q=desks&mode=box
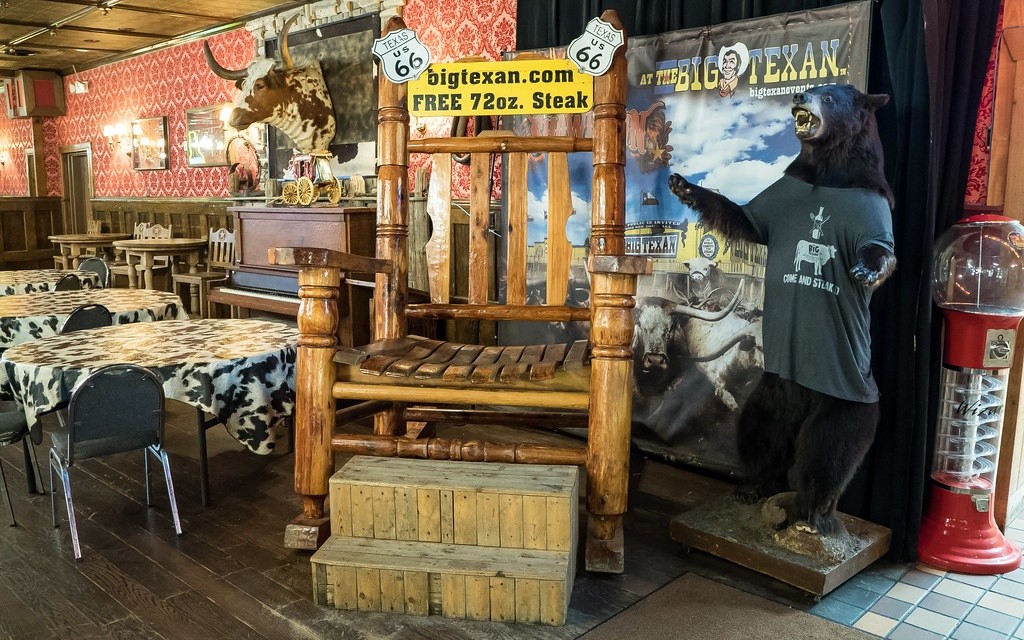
[48,234,132,270]
[112,238,207,317]
[0,288,190,401]
[0,269,104,296]
[2,319,300,507]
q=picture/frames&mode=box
[129,117,170,171]
[185,103,268,167]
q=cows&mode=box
[500,258,765,414]
[203,13,336,151]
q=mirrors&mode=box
[264,11,380,180]
[0,75,38,197]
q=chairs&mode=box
[284,9,637,573]
[171,228,236,318]
[52,219,102,270]
[0,258,182,563]
[109,222,172,291]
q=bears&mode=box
[667,84,896,541]
